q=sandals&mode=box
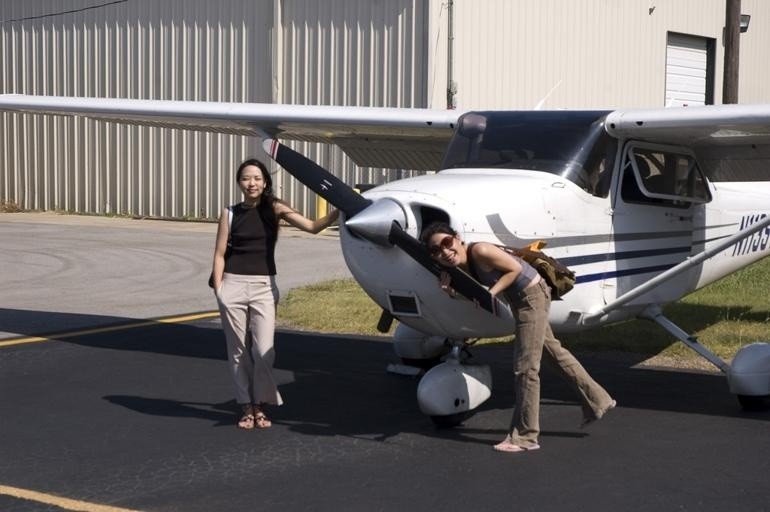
[237,407,273,430]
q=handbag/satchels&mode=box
[207,204,235,288]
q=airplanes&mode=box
[0,97,770,428]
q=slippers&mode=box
[494,437,541,453]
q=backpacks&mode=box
[467,238,577,302]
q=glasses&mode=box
[428,233,456,258]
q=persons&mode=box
[212,158,341,430]
[422,222,617,454]
